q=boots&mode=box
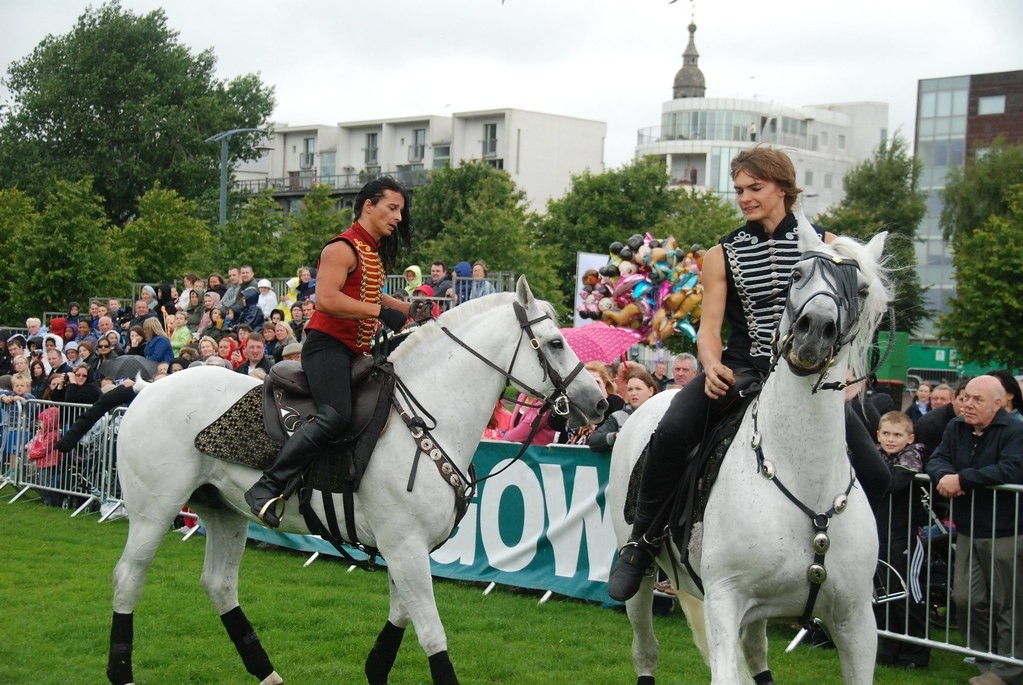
[243,417,328,528]
[608,498,673,602]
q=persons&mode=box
[485,352,1023,685]
[607,147,892,601]
[0,261,495,549]
[750,122,756,142]
[689,166,697,185]
[243,175,429,527]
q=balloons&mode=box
[578,232,707,346]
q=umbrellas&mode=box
[558,321,640,365]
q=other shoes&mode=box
[968,672,1008,685]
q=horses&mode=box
[606,204,909,685]
[106,273,609,685]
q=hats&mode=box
[282,343,303,356]
[65,341,79,352]
[258,279,272,289]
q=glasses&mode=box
[98,345,110,349]
[76,374,88,378]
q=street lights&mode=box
[205,124,276,266]
